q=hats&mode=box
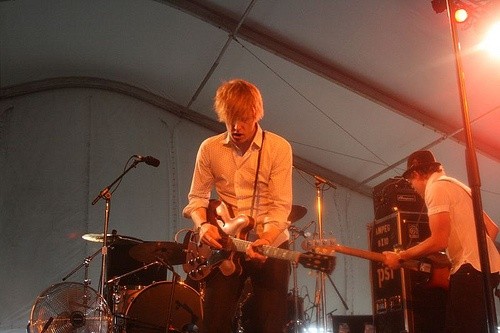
[402,150,442,179]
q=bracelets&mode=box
[396,249,406,263]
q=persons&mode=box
[381,150,500,333]
[182,79,292,333]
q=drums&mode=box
[115,280,204,333]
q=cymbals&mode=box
[287,205,307,223]
[128,240,184,265]
[82,231,144,245]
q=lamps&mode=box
[431,0,447,15]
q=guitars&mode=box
[183,198,338,278]
[299,237,453,288]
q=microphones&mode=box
[315,175,337,189]
[134,155,160,167]
[289,221,313,245]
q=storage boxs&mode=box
[365,208,449,333]
[370,175,435,221]
